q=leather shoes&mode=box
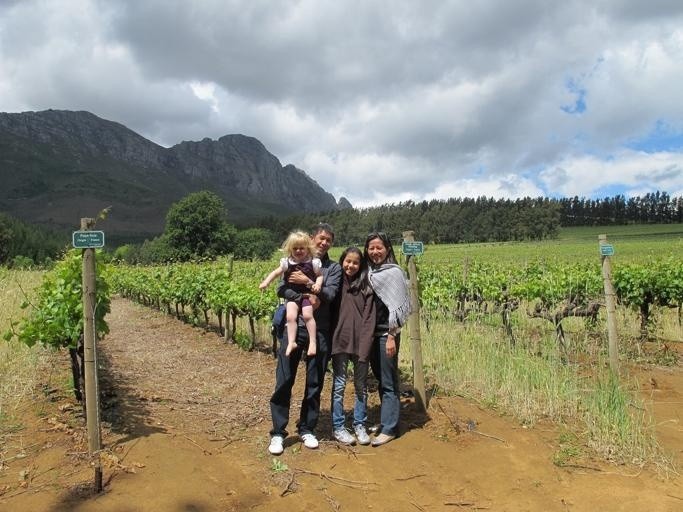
[368,424,397,447]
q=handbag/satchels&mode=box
[272,303,286,339]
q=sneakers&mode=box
[301,433,320,449]
[333,429,356,446]
[268,436,285,455]
[355,425,371,445]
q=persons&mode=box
[330,247,377,445]
[268,222,342,455]
[360,232,412,445]
[259,231,324,357]
